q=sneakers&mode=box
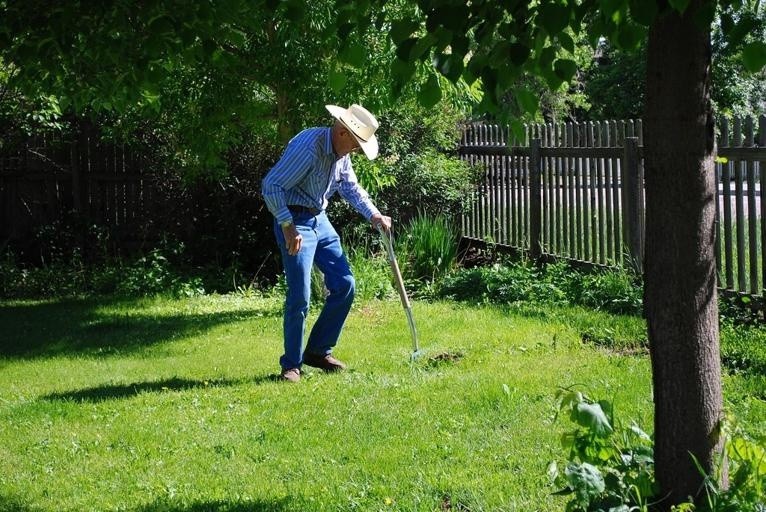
[282,368,301,383]
[302,351,347,371]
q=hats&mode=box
[325,103,380,161]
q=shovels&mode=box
[374,223,420,362]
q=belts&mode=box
[286,204,316,212]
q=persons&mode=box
[261,99,395,382]
